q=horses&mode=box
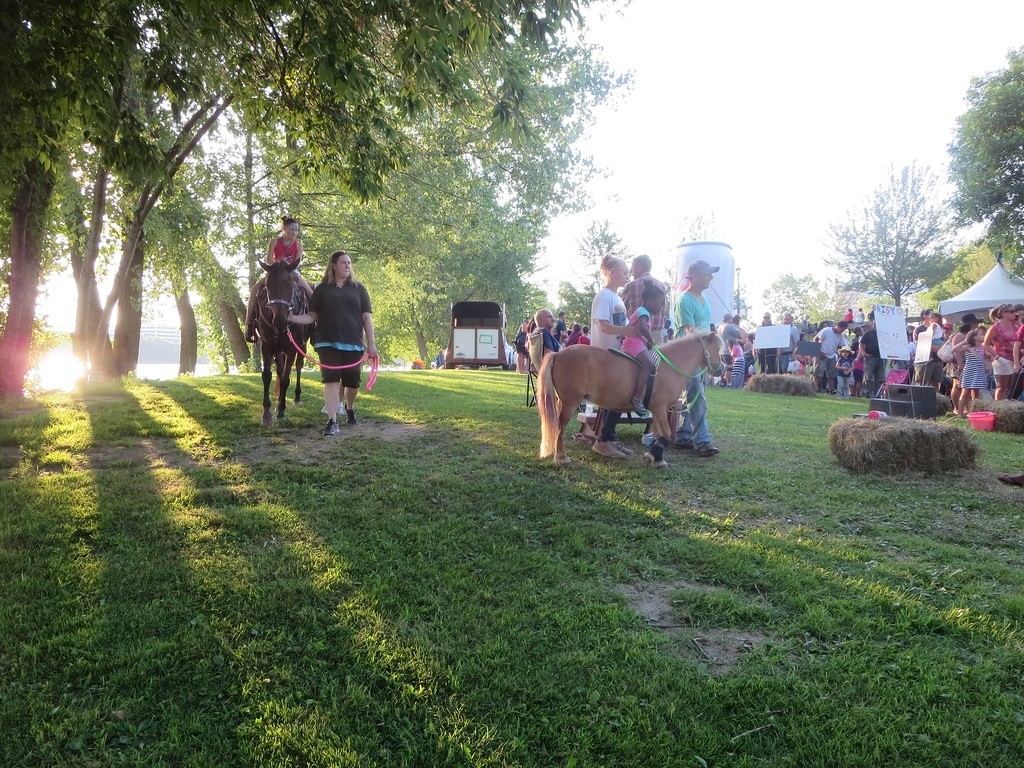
[532,322,725,468]
[248,257,316,426]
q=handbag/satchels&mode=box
[936,338,957,362]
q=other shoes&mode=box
[714,377,751,393]
[814,387,885,398]
[950,408,977,420]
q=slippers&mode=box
[998,475,1023,488]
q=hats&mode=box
[958,313,983,328]
[722,313,733,321]
[1012,303,1024,311]
[685,258,720,278]
[837,345,853,354]
[597,252,623,274]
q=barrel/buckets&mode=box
[967,411,997,432]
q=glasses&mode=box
[1004,310,1015,313]
[923,307,932,314]
[763,316,769,319]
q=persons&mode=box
[949,313,984,416]
[532,310,562,353]
[621,255,666,346]
[984,302,1024,401]
[436,350,444,367]
[513,318,526,372]
[588,254,643,456]
[665,318,716,343]
[245,215,315,326]
[527,317,536,332]
[719,307,952,396]
[622,279,664,417]
[673,261,720,458]
[998,472,1024,487]
[1012,304,1024,322]
[552,311,590,348]
[952,329,989,420]
[1007,323,1024,403]
[287,252,378,436]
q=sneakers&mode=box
[337,401,344,415]
[592,438,631,459]
[320,404,327,415]
[323,419,340,434]
[674,436,694,450]
[696,442,720,456]
[343,405,356,425]
